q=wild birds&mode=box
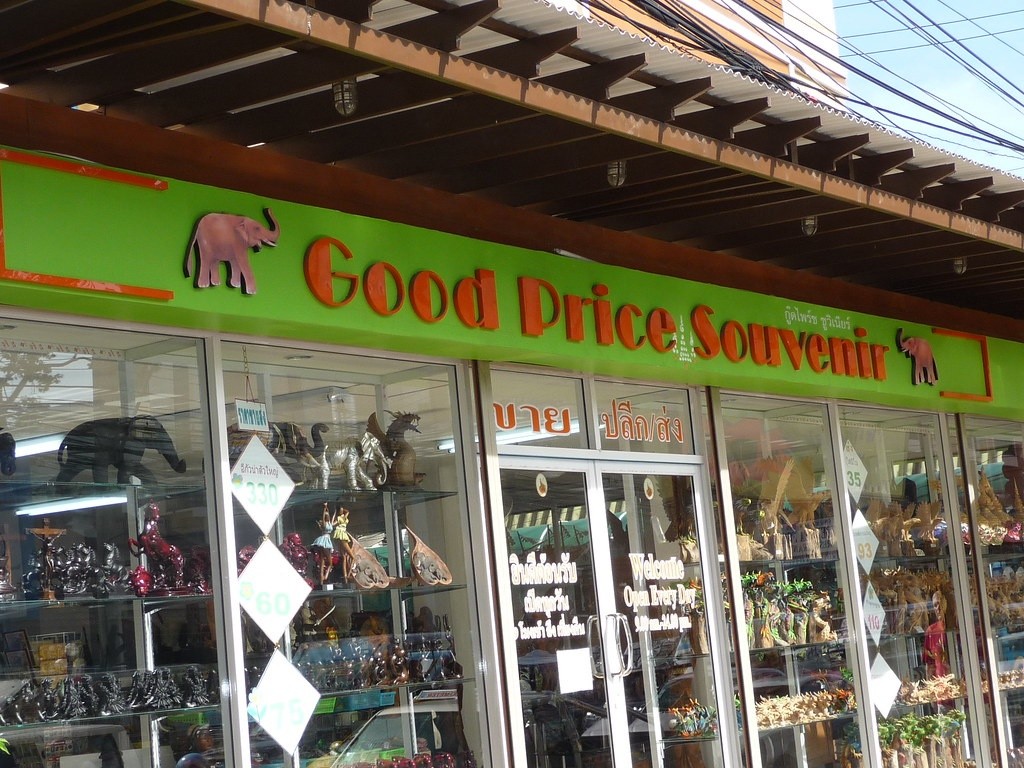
[738,454,945,544]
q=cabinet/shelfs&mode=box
[643,554,1024,768]
[0,483,477,768]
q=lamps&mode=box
[893,450,1003,477]
[14,493,128,516]
[801,216,818,239]
[437,421,606,454]
[154,386,349,421]
[333,77,358,117]
[607,161,626,188]
[954,258,967,274]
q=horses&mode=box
[20,498,213,599]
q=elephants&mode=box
[897,327,939,386]
[184,208,281,295]
[55,414,187,496]
[226,421,391,491]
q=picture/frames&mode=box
[2,629,36,670]
